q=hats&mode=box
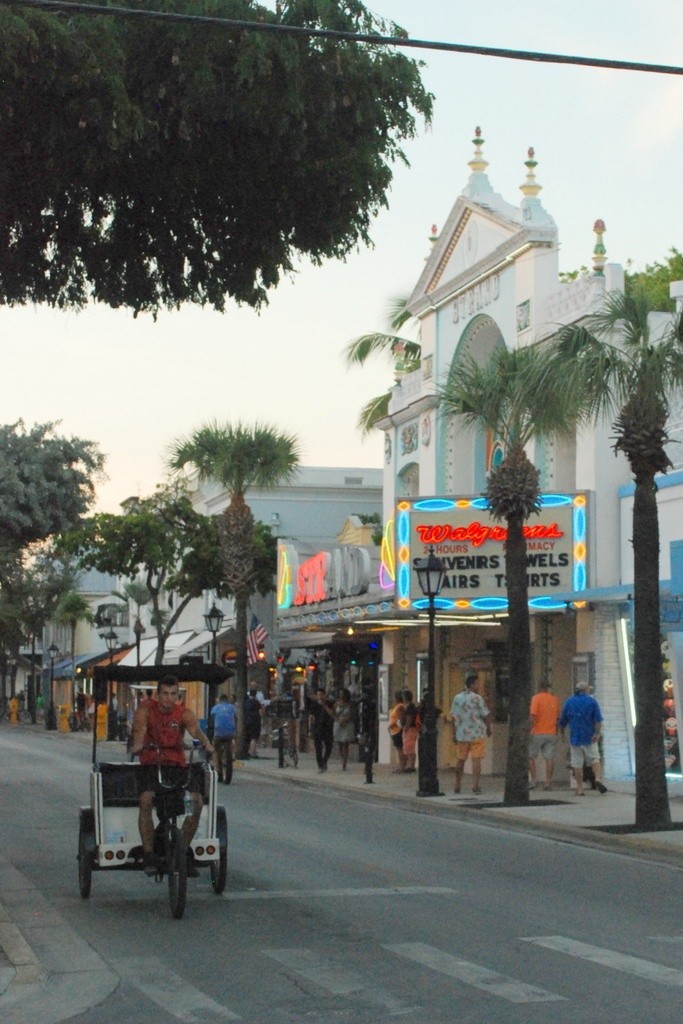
[576,682,593,691]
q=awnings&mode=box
[52,624,233,682]
[279,633,365,648]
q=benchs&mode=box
[99,761,206,807]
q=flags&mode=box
[246,614,268,667]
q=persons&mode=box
[450,675,492,795]
[400,691,416,773]
[528,680,561,790]
[334,688,356,770]
[129,676,215,878]
[387,691,408,774]
[560,681,607,796]
[307,688,336,773]
[14,690,264,759]
[415,687,444,732]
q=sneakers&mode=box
[144,854,156,875]
[217,755,609,796]
[186,857,199,877]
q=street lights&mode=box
[47,643,60,729]
[202,603,227,741]
[411,544,450,797]
[103,624,119,742]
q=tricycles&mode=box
[75,661,236,920]
